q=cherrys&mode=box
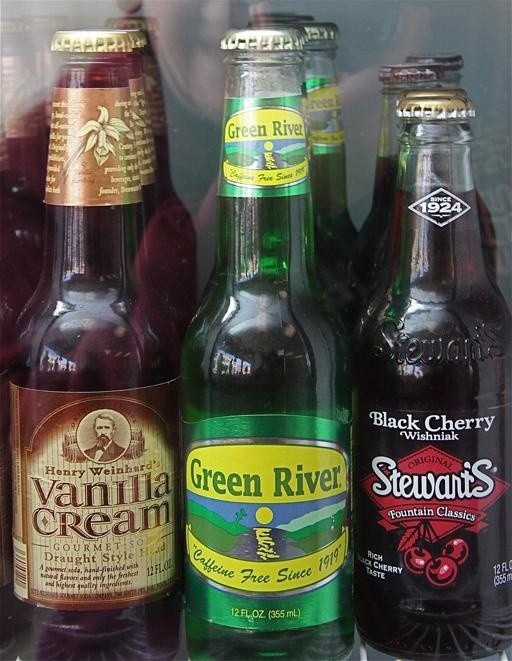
[403,521,469,585]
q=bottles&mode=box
[358,64,441,336]
[1,30,186,661]
[107,16,200,313]
[123,30,185,365]
[358,86,498,351]
[180,25,354,661]
[406,54,497,281]
[253,12,362,319]
[351,94,512,661]
